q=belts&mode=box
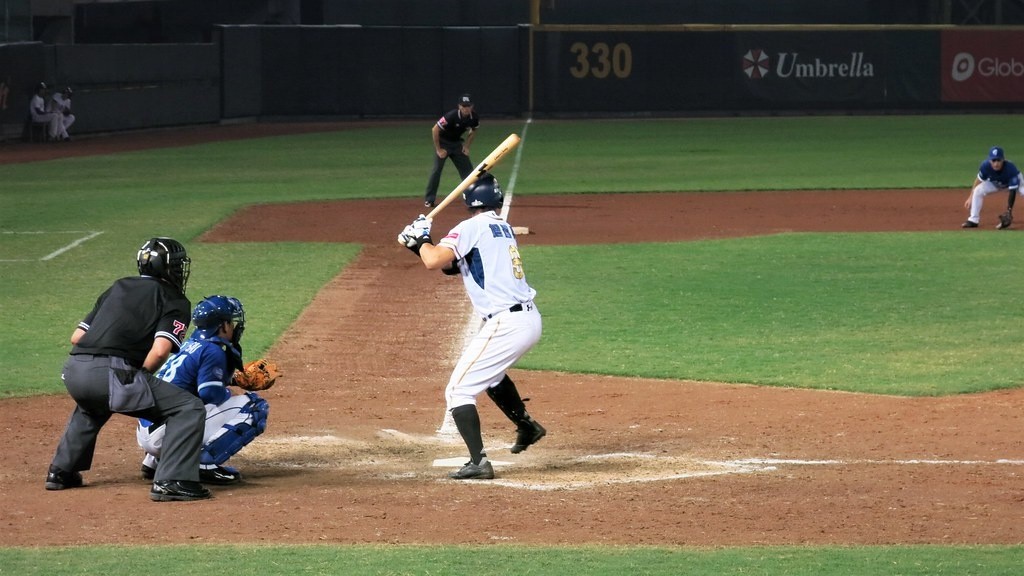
[483,304,529,321]
[137,422,163,434]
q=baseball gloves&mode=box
[235,359,283,391]
[996,210,1012,230]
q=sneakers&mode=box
[46,464,82,490]
[510,420,546,454]
[149,480,210,502]
[199,466,236,485]
[141,464,156,480]
[451,461,494,479]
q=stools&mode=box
[29,121,48,143]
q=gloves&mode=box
[413,213,434,248]
[397,224,420,256]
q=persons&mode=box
[960,146,1024,229]
[29,81,64,144]
[135,295,269,486]
[47,86,76,143]
[45,239,212,502]
[424,95,479,207]
[398,173,547,479]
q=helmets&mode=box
[463,173,503,209]
[137,237,191,296]
[193,296,233,337]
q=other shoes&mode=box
[425,201,434,207]
[961,221,978,228]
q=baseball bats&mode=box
[398,133,521,246]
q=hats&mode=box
[989,147,1004,160]
[458,94,473,107]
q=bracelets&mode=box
[417,234,433,249]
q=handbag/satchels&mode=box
[108,356,156,412]
